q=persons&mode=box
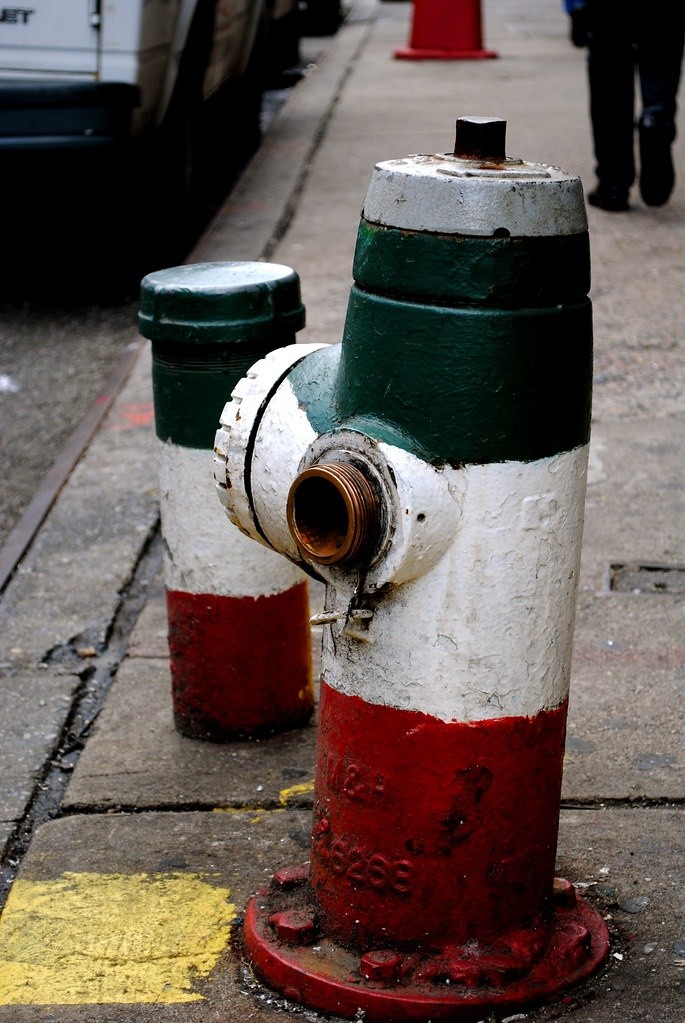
[559,0,685,211]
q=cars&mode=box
[1,1,350,306]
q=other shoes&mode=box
[586,187,631,212]
[640,116,676,208]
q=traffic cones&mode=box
[393,1,502,62]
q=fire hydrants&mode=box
[210,112,610,1023]
